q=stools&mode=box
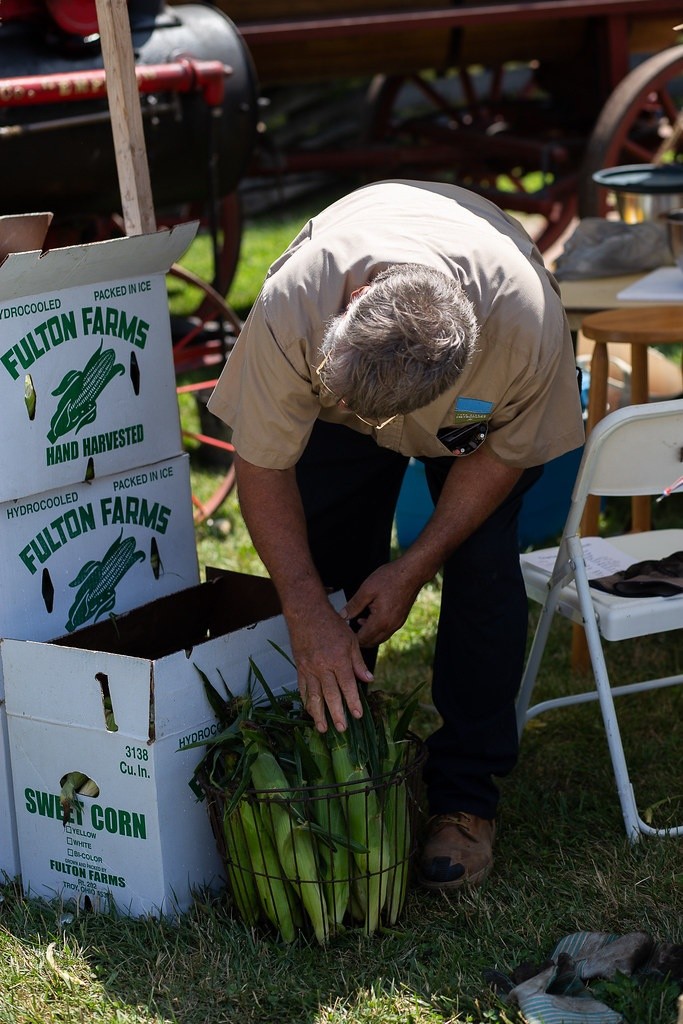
[570,305,683,671]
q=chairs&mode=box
[517,398,683,846]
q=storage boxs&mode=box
[1,209,351,926]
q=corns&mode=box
[60,692,117,796]
[226,703,410,944]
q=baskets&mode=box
[197,729,430,950]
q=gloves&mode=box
[485,930,683,1024]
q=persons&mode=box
[207,178,586,891]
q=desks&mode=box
[554,269,683,359]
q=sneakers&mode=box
[418,810,502,889]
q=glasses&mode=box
[316,346,400,431]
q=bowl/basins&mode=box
[657,208,683,267]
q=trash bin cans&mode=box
[592,163,683,259]
